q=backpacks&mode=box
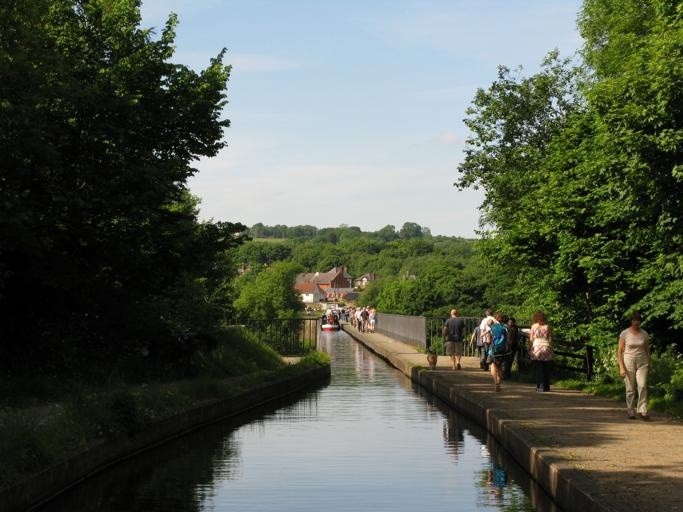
[491,327,507,354]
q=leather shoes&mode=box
[639,414,648,419]
[628,416,634,419]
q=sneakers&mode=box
[456,363,460,370]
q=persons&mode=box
[306,304,377,333]
[470,310,518,392]
[442,309,467,369]
[527,311,554,394]
[618,311,653,421]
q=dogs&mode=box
[427,347,437,371]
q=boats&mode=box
[320,324,338,331]
